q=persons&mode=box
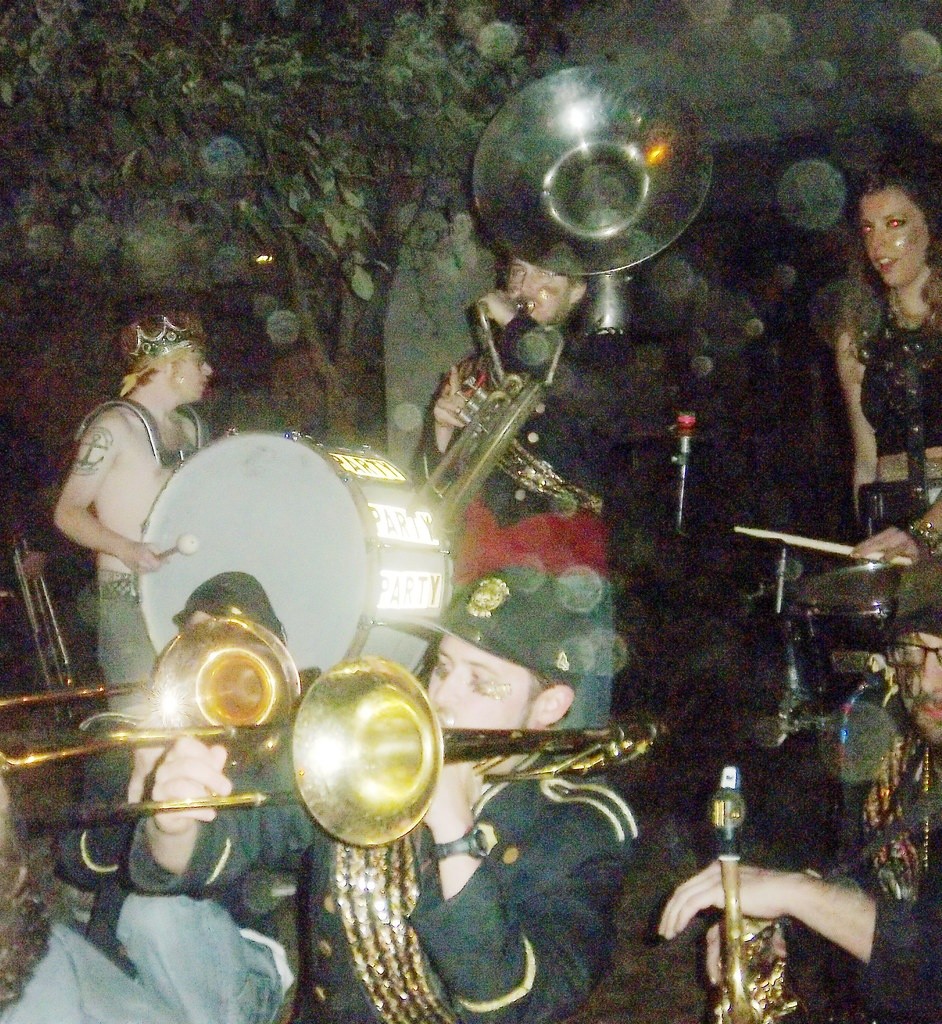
[130,563,639,1024]
[655,607,942,1024]
[52,305,216,724]
[818,164,942,568]
[408,238,629,778]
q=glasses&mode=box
[883,640,940,669]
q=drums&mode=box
[797,557,942,721]
[136,425,452,683]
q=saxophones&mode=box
[710,766,806,1022]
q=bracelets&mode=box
[906,514,942,556]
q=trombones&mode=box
[10,530,82,728]
[0,620,319,776]
[89,657,671,844]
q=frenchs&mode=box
[408,69,720,533]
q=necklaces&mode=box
[888,289,933,327]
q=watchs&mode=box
[428,827,491,861]
[0,570,290,1021]
